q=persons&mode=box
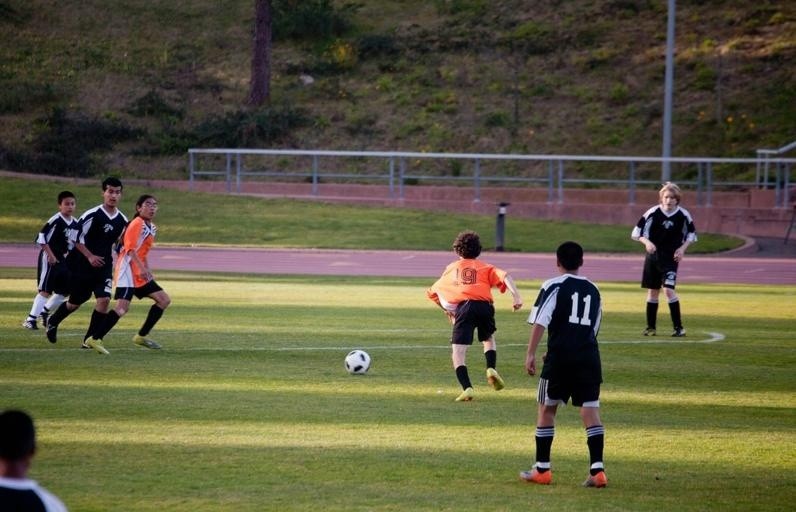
[84,193,170,354]
[44,177,127,347]
[630,181,697,339]
[22,191,77,330]
[0,409,68,512]
[425,229,522,403]
[518,242,609,488]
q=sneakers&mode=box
[671,328,687,337]
[455,387,475,402]
[519,465,553,485]
[82,337,110,356]
[486,368,506,393]
[40,312,48,329]
[22,318,38,331]
[132,333,163,352]
[45,315,58,344]
[642,328,657,337]
[581,470,609,487]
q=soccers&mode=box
[344,350,371,374]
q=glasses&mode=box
[141,204,158,208]
